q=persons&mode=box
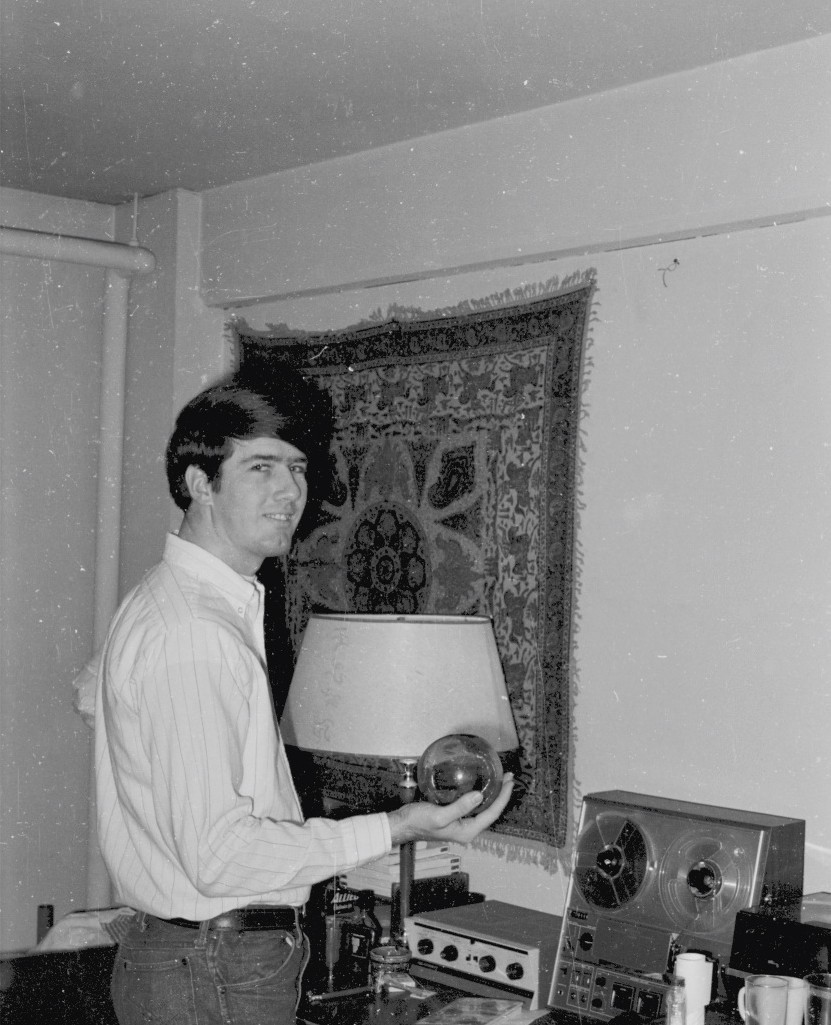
[96,380,513,1025]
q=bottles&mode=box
[664,976,687,1025]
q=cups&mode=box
[738,975,809,1025]
[803,973,831,1025]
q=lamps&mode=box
[278,613,517,945]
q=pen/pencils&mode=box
[312,985,375,1002]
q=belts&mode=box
[157,907,304,931]
